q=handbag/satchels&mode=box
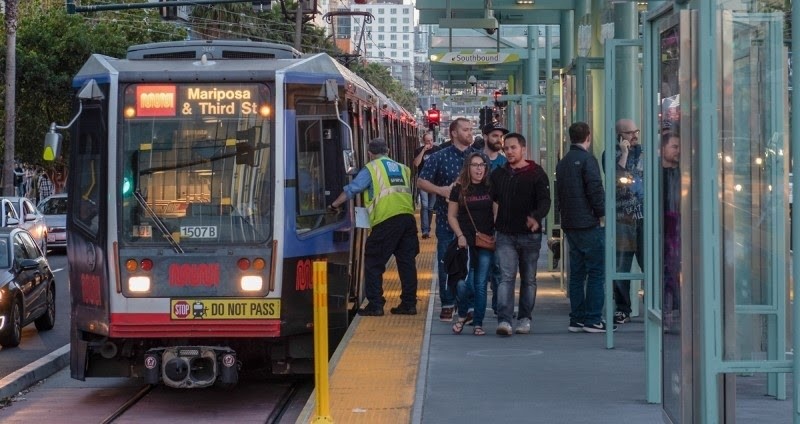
[615,212,637,251]
[476,232,496,251]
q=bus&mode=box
[44,11,420,392]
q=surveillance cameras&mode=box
[483,10,498,35]
[468,75,478,87]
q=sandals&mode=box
[452,319,465,333]
[472,327,486,335]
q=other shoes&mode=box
[583,321,618,332]
[357,303,384,316]
[390,303,417,315]
[614,311,630,324]
[440,307,455,321]
[515,318,531,333]
[496,322,512,335]
[568,321,584,332]
[422,231,429,239]
[465,311,474,325]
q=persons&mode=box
[414,117,553,338]
[601,119,644,325]
[555,121,618,334]
[659,126,680,333]
[327,137,420,317]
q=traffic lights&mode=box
[494,89,507,107]
[428,111,439,123]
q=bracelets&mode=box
[331,204,337,209]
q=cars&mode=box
[0,226,56,348]
[37,193,69,255]
[0,196,48,261]
[716,136,794,253]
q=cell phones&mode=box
[617,134,625,143]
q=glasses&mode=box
[470,162,487,168]
[621,129,640,136]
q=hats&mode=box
[482,122,509,135]
